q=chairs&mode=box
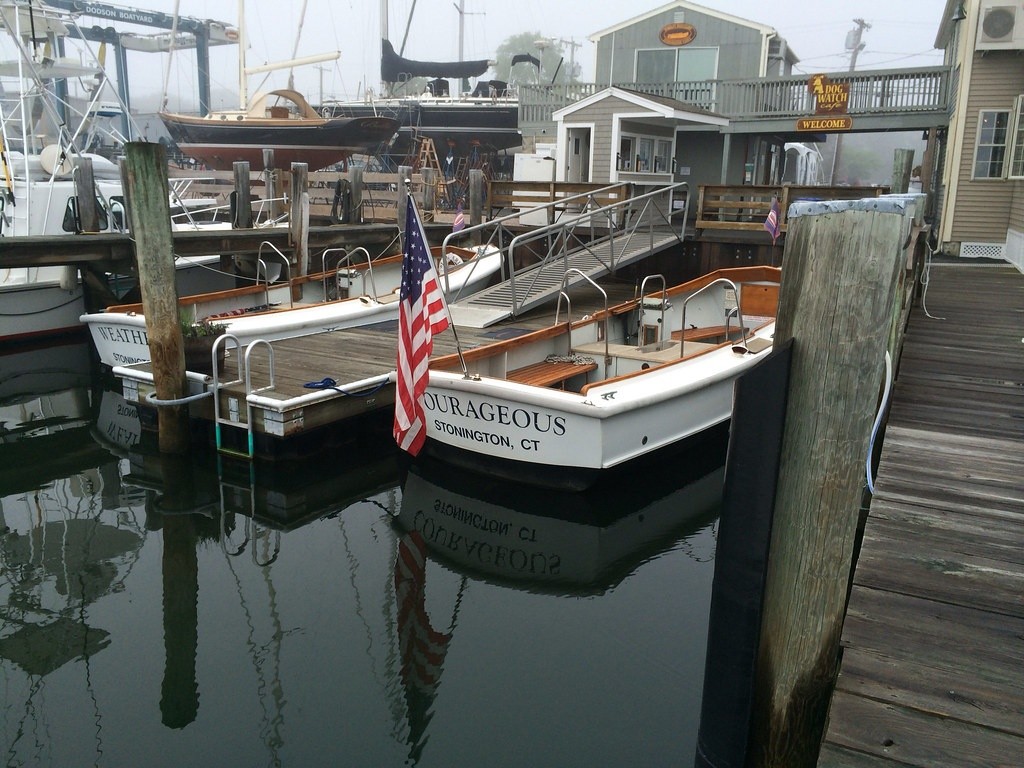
[426,78,449,97]
[471,79,508,98]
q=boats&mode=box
[418,266,783,471]
[0,336,156,679]
[158,0,523,188]
[391,438,727,597]
[82,246,507,402]
[1,0,297,349]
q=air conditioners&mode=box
[974,0,1024,59]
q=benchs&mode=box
[506,360,600,391]
[670,326,750,344]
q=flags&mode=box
[452,204,465,235]
[392,195,451,456]
[764,197,780,244]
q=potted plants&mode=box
[177,305,228,377]
